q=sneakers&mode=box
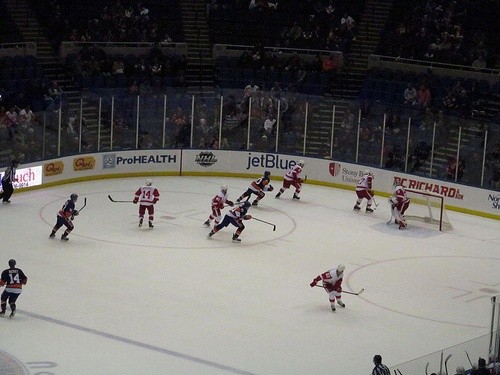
[148,221,154,229]
[137,219,143,227]
[365,207,374,214]
[235,199,240,203]
[0,310,6,318]
[275,192,281,199]
[337,300,345,308]
[206,233,212,240]
[353,204,361,211]
[292,193,300,201]
[8,313,15,320]
[48,233,56,239]
[61,234,69,242]
[252,203,258,207]
[231,233,241,243]
[331,305,336,312]
[204,221,210,227]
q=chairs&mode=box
[0,0,500,184]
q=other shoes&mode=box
[3,200,10,204]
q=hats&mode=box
[244,201,251,207]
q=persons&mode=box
[0,259,27,320]
[397,0,500,72]
[0,82,93,165]
[238,171,274,206]
[276,160,305,200]
[46,0,172,48]
[50,193,79,240]
[207,0,356,51]
[75,47,337,154]
[205,187,234,227]
[394,179,410,229]
[210,202,252,242]
[310,264,345,311]
[470,358,490,375]
[0,159,19,202]
[354,170,374,213]
[372,355,390,375]
[340,68,500,188]
[133,179,160,229]
[456,366,466,375]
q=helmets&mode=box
[8,259,16,267]
[220,185,227,192]
[145,179,153,186]
[298,159,304,165]
[70,194,78,200]
[337,265,345,273]
[264,171,270,177]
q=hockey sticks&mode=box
[424,361,430,375]
[444,353,453,375]
[439,350,444,375]
[251,217,276,232]
[465,349,474,368]
[303,175,308,182]
[223,195,251,207]
[76,197,87,214]
[314,284,365,296]
[4,282,7,285]
[369,188,379,207]
[393,369,397,375]
[396,368,403,375]
[108,195,140,202]
